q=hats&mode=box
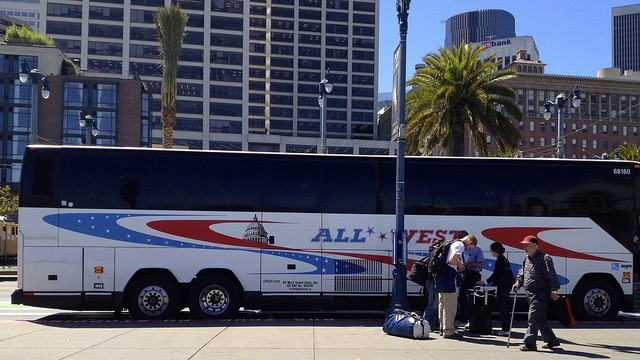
[520,235,538,245]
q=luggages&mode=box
[469,282,492,334]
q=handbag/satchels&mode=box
[406,262,429,286]
[555,295,576,327]
[383,309,430,339]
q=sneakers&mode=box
[542,339,560,348]
[443,332,463,338]
[520,344,537,351]
[497,330,509,336]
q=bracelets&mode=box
[551,289,558,294]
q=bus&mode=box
[12,146,640,321]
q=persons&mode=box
[436,234,479,339]
[457,230,484,327]
[512,236,561,351]
[422,239,443,332]
[476,242,516,336]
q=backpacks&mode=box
[428,239,461,273]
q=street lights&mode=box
[79,111,98,146]
[317,67,334,153]
[18,57,50,145]
[544,85,582,158]
